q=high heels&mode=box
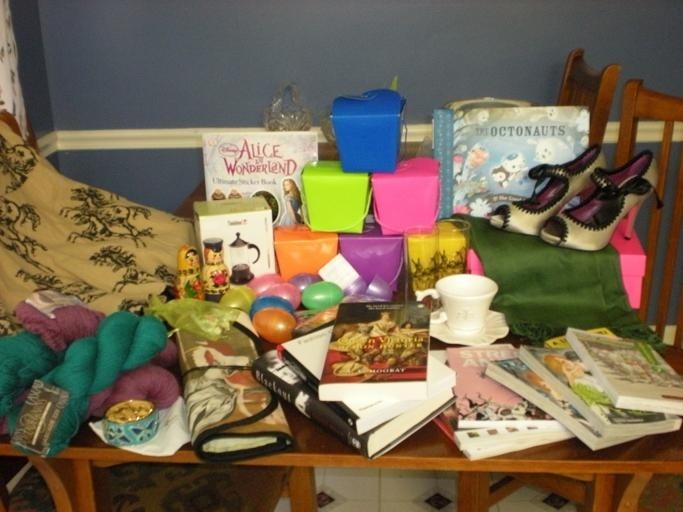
[489,143,606,236]
[539,149,659,252]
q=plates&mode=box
[427,308,509,346]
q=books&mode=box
[202,129,318,227]
[432,105,592,219]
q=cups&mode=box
[437,217,471,277]
[404,226,437,304]
[415,274,498,336]
[227,233,260,286]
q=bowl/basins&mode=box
[101,398,159,444]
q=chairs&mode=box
[380,46,682,373]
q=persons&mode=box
[281,178,305,226]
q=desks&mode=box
[1,401,683,511]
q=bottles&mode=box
[263,79,310,132]
